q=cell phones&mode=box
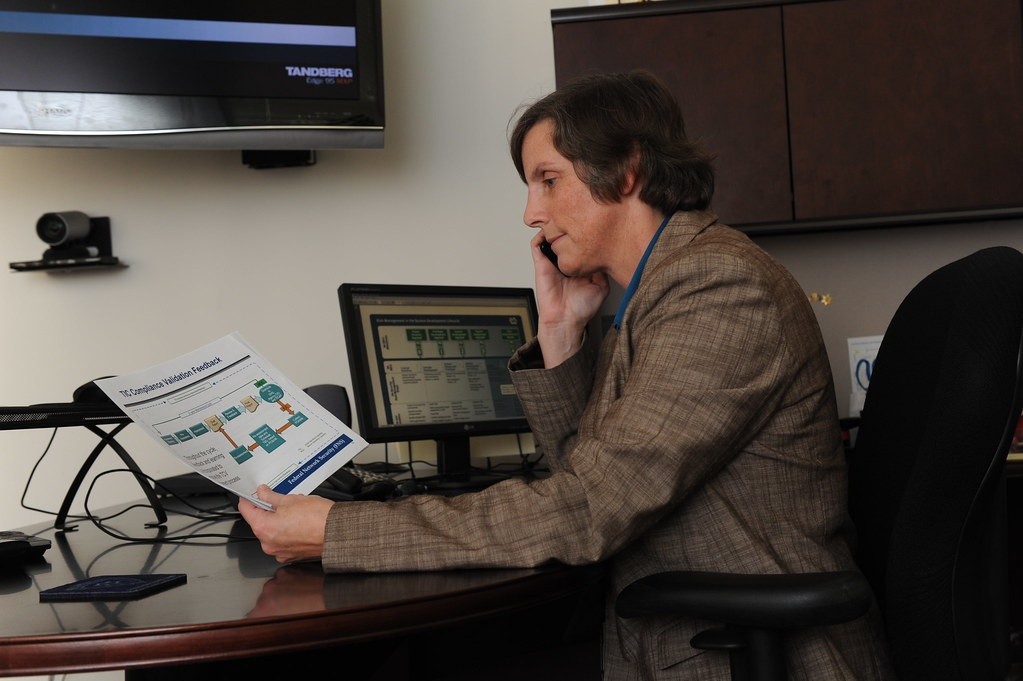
[541,241,569,279]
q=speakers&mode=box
[301,385,352,431]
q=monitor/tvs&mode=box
[340,283,539,497]
[0,0,389,152]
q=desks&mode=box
[0,453,608,681]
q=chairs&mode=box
[615,244,1022,680]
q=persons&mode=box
[238,70,884,680]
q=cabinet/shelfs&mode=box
[551,0,1022,231]
